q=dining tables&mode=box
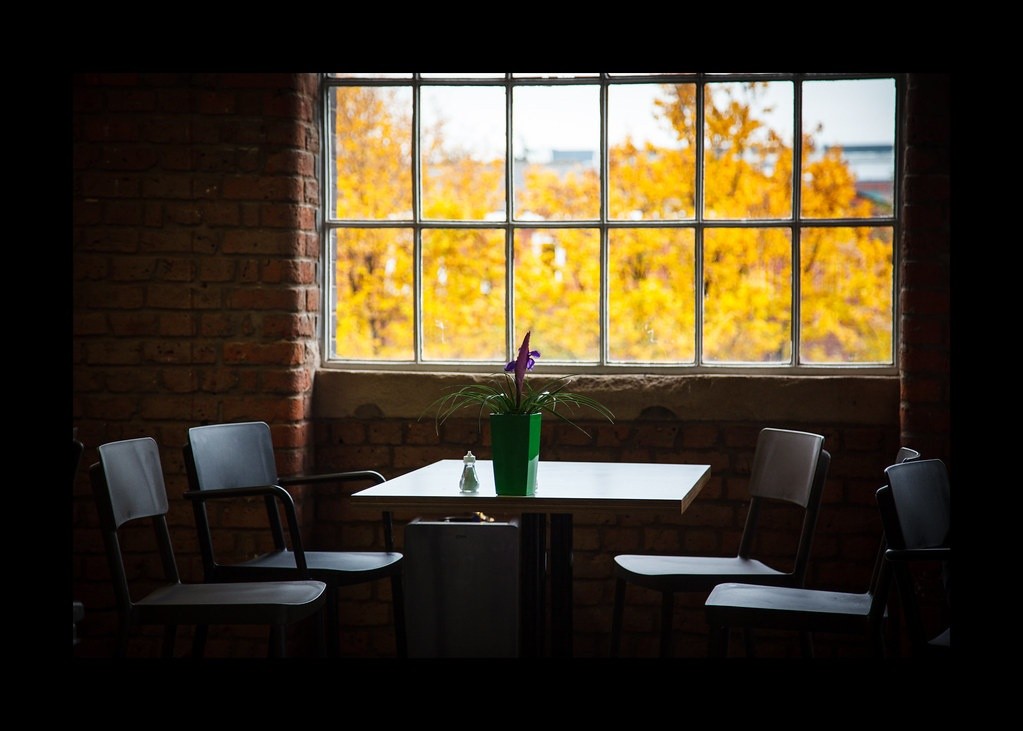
[350,458,712,731]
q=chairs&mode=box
[704,447,921,731]
[875,459,1023,731]
[182,421,410,714]
[608,427,832,717]
[90,438,325,731]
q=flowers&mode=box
[417,331,616,438]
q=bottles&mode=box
[458,450,480,492]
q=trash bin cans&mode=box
[403,514,522,659]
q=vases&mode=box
[490,412,541,496]
